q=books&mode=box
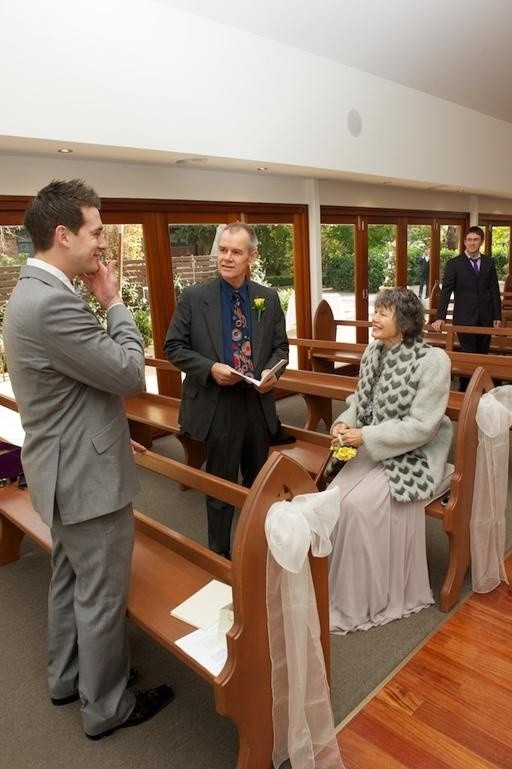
[229,358,287,389]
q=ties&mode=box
[231,289,255,379]
[470,257,480,278]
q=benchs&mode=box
[124,356,495,615]
[275,285,511,428]
[1,392,332,768]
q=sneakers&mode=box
[217,550,231,561]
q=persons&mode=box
[0,177,177,741]
[417,247,431,299]
[430,226,502,394]
[323,285,455,636]
[157,219,291,554]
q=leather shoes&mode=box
[85,683,176,741]
[51,667,140,707]
